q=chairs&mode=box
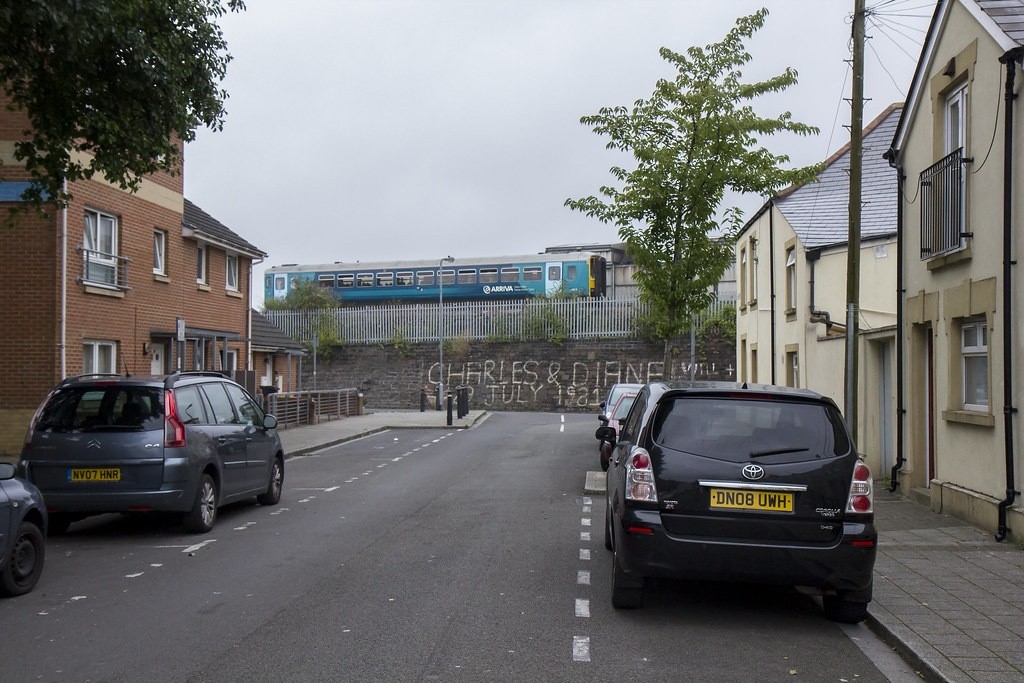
[116,402,144,426]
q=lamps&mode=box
[143,342,150,355]
[264,352,270,366]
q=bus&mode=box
[262,251,608,310]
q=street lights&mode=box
[439,256,456,407]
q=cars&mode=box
[600,384,649,428]
[598,392,639,454]
[0,462,48,594]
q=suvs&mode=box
[595,380,879,626]
[20,373,285,537]
